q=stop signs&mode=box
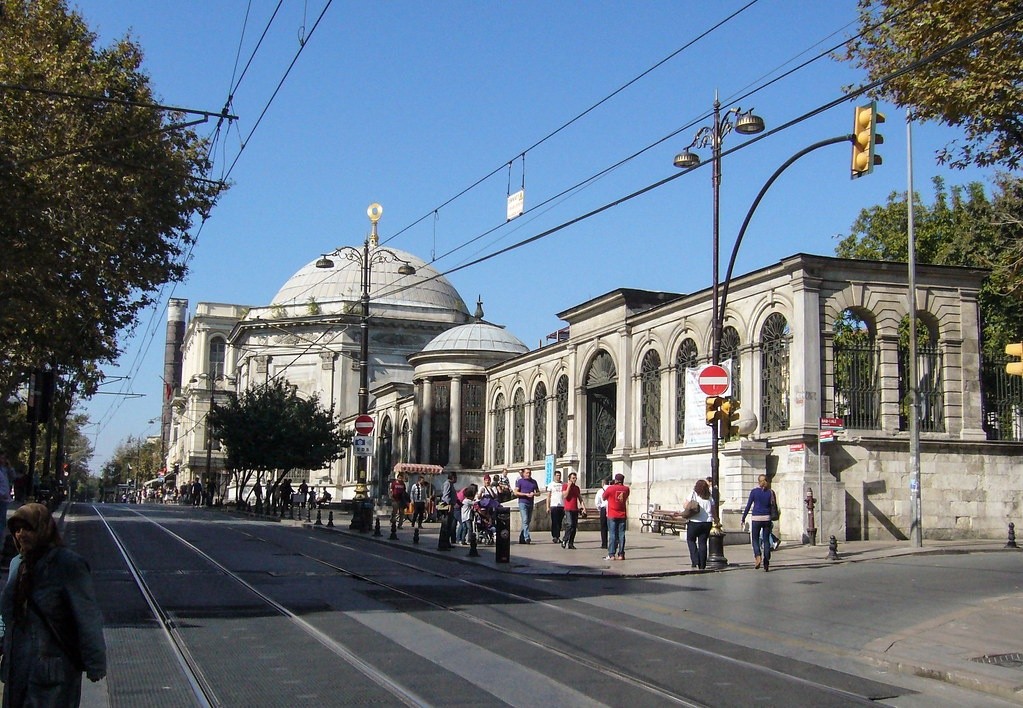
[699,365,730,396]
[354,415,374,436]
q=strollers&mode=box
[473,506,496,545]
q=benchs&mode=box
[576,507,601,530]
[316,497,332,509]
[639,510,688,536]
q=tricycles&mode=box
[394,463,447,523]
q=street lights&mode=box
[316,233,417,532]
[673,89,764,569]
[147,413,179,504]
[189,367,237,511]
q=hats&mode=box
[484,474,491,482]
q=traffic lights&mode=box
[1006,341,1023,378]
[850,101,885,180]
[705,396,722,424]
[719,396,741,442]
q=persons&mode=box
[120,478,217,510]
[410,477,436,529]
[682,481,717,569]
[266,479,296,510]
[298,479,309,509]
[254,480,263,504]
[546,471,565,543]
[595,479,609,548]
[560,472,586,549]
[482,465,483,469]
[452,466,512,546]
[0,503,107,708]
[390,475,406,531]
[705,477,712,494]
[601,473,630,560]
[0,451,12,580]
[514,468,541,544]
[307,485,316,509]
[438,472,464,550]
[740,474,779,573]
[319,486,328,506]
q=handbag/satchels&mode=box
[435,501,451,522]
[770,490,779,521]
[682,492,700,518]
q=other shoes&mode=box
[552,537,562,544]
[519,539,531,544]
[561,540,566,548]
[568,545,577,549]
[603,555,615,560]
[619,555,625,560]
[763,564,768,572]
[755,555,761,569]
[418,525,423,529]
[397,525,404,530]
